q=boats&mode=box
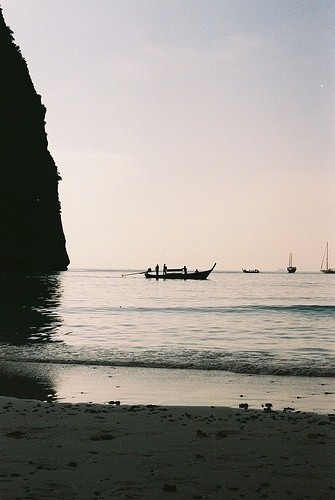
[242,269,259,273]
[144,263,217,280]
[166,269,183,271]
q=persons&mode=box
[155,264,159,275]
[195,268,200,273]
[182,266,188,274]
[162,264,167,275]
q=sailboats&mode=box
[286,253,296,273]
[319,242,335,273]
